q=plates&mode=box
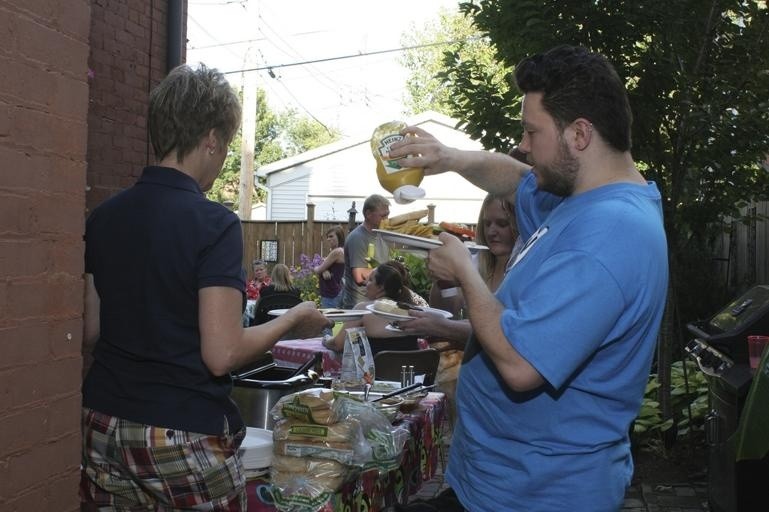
[266,308,371,323]
[370,227,491,255]
[238,427,275,470]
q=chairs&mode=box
[253,295,303,326]
[374,347,440,392]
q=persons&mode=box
[82,58,338,512]
[395,193,519,353]
[386,43,671,512]
[322,260,431,356]
[245,260,300,326]
[314,194,391,307]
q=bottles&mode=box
[370,120,426,206]
[400,366,416,388]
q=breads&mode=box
[272,393,347,493]
[380,207,434,239]
[374,298,409,316]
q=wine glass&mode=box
[366,303,453,322]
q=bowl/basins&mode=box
[343,387,429,423]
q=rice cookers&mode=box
[228,363,319,434]
